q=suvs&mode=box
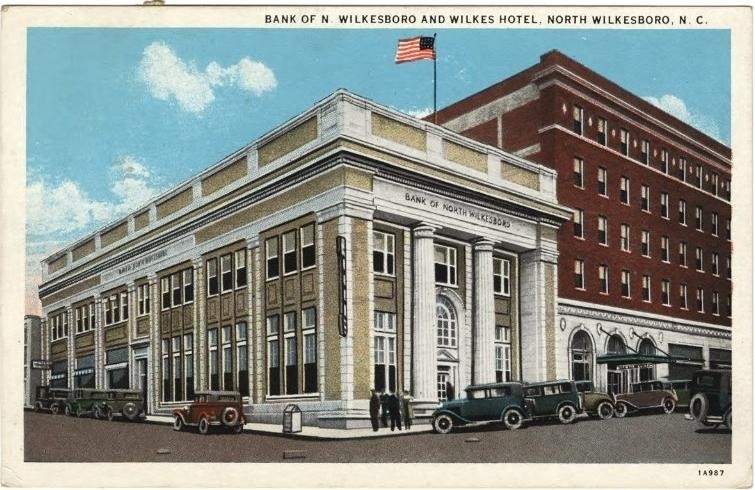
[432,379,618,434]
[615,368,731,431]
[34,388,147,423]
[173,391,248,435]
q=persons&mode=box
[369,389,380,432]
[400,389,416,430]
[379,388,390,428]
[388,386,402,431]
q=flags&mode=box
[394,35,437,64]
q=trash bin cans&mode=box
[283,404,302,434]
